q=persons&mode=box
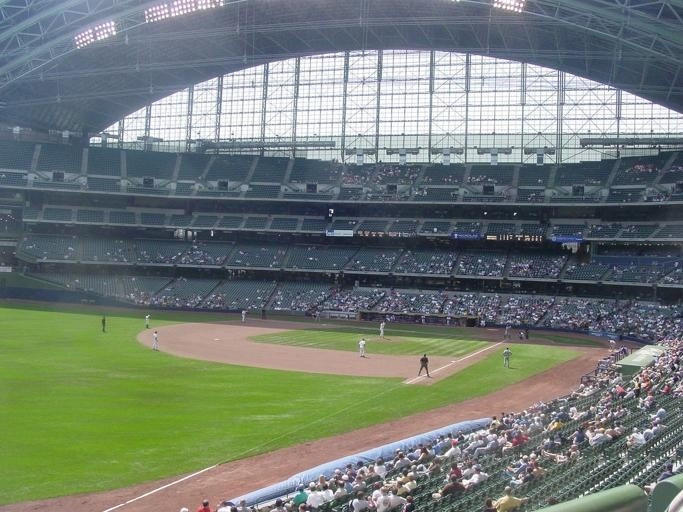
[1,158,682,340]
[153,331,159,351]
[182,339,682,512]
